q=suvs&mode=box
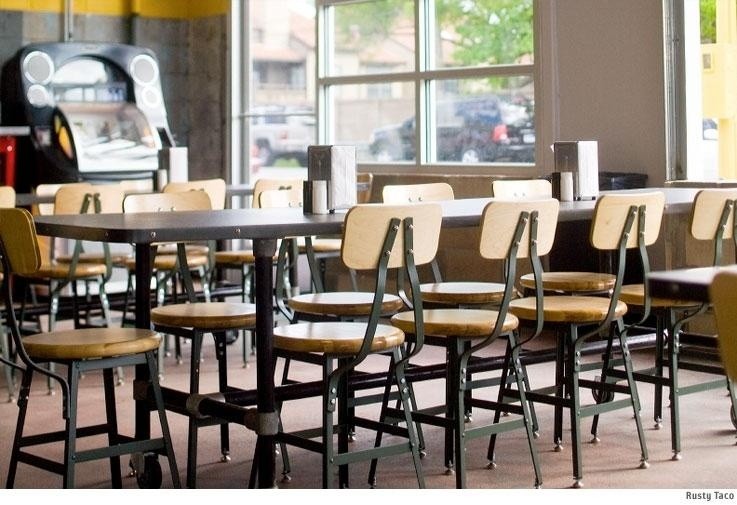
[370,95,535,163]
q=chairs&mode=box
[0,171,737,489]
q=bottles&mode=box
[152,169,168,193]
[303,180,329,214]
[552,171,574,202]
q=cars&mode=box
[250,105,317,166]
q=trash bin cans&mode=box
[599,171,646,190]
